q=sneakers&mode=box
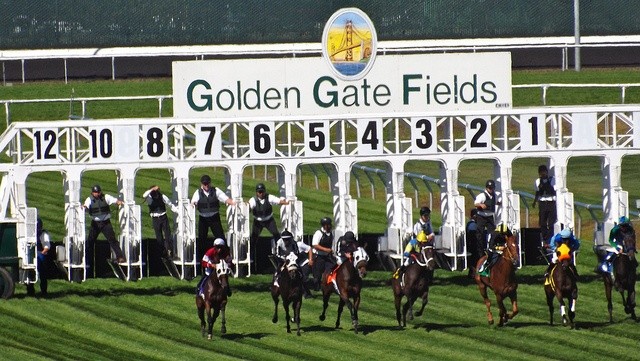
[118,257,126,263]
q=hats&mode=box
[280,231,293,239]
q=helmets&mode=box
[619,216,629,224]
[37,218,43,228]
[486,180,495,187]
[256,183,266,192]
[321,217,332,225]
[345,232,355,241]
[201,175,211,184]
[213,238,225,246]
[562,228,572,239]
[420,207,430,215]
[538,165,547,172]
[93,186,101,192]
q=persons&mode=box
[312,217,334,291]
[531,165,557,245]
[272,228,300,285]
[487,221,513,268]
[199,238,230,298]
[294,233,313,298]
[83,185,125,262]
[544,227,579,277]
[191,174,236,260]
[28,219,51,297]
[474,180,496,252]
[601,216,631,272]
[397,231,431,276]
[336,231,359,264]
[245,183,289,262]
[144,185,179,256]
[403,208,433,238]
[467,209,477,231]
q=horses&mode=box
[390,235,435,330]
[268,246,306,337]
[195,255,233,342]
[316,242,372,335]
[599,229,640,326]
[473,231,521,328]
[543,238,579,329]
